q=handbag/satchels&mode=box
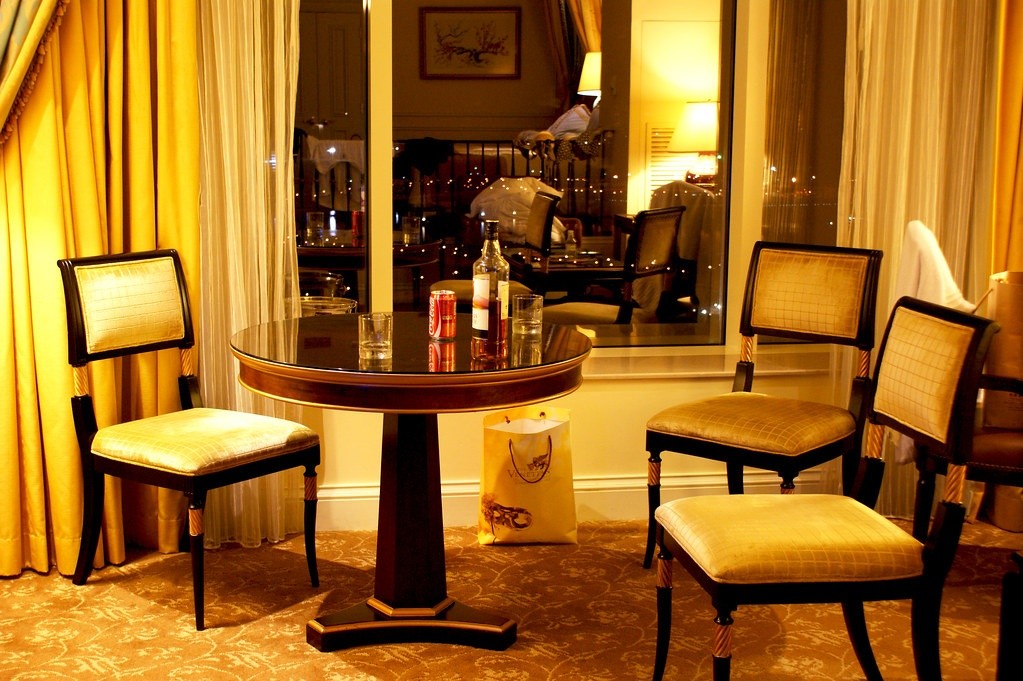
[477,406,578,544]
[983,271,1023,429]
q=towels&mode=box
[633,179,718,322]
[890,219,977,469]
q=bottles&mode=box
[565,230,576,258]
[473,220,510,341]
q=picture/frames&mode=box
[418,5,525,81]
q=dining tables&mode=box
[227,308,593,652]
[294,226,447,312]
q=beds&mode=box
[308,134,571,234]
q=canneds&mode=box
[427,343,456,371]
[428,289,458,340]
[352,211,366,246]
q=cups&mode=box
[512,293,544,335]
[358,313,394,360]
[402,217,420,246]
[305,211,324,238]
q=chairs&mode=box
[639,240,885,572]
[647,295,1004,681]
[542,204,690,324]
[911,271,1023,556]
[427,189,564,315]
[55,247,323,631]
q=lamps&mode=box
[666,100,724,186]
[575,50,605,112]
